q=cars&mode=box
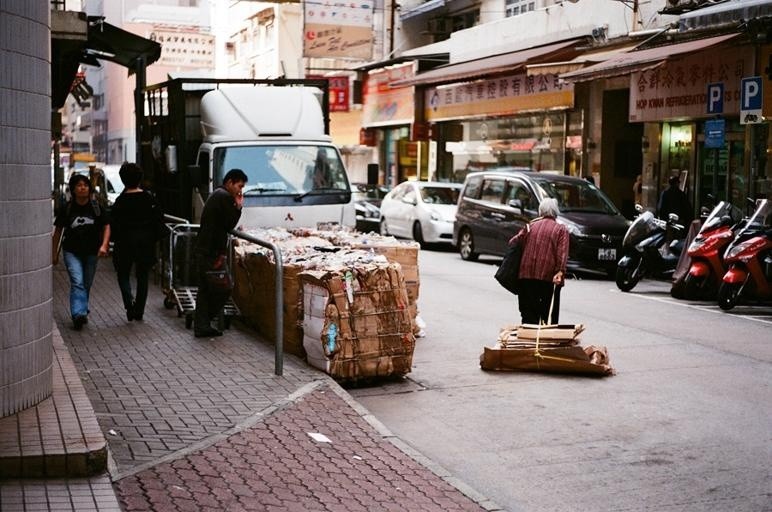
[378,180,464,248]
[350,182,387,231]
[50,152,126,210]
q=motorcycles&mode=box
[615,196,772,311]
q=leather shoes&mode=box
[194,328,223,338]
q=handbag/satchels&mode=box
[152,200,166,241]
[494,236,526,296]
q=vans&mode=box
[450,165,631,278]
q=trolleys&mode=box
[162,222,242,330]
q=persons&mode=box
[658,175,693,227]
[109,162,164,323]
[632,173,642,206]
[51,174,112,327]
[192,168,249,339]
[507,196,571,327]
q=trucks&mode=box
[130,74,356,238]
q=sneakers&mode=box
[126,310,143,321]
[71,310,91,331]
